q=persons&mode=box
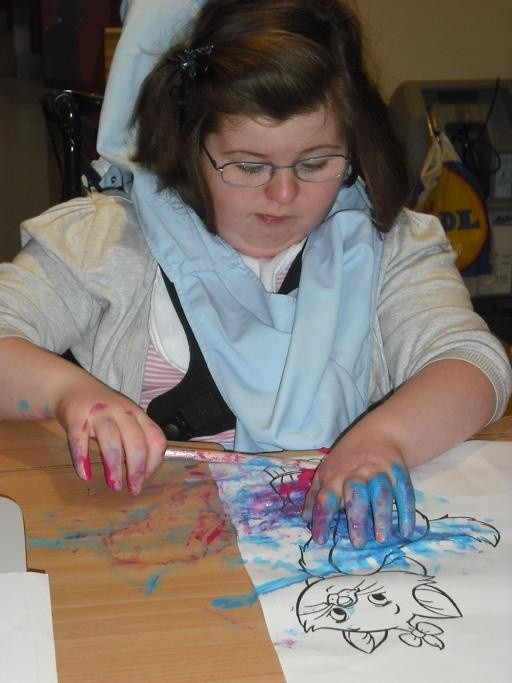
[0,0,510,547]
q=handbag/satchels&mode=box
[406,132,493,278]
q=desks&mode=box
[0,406,512,683]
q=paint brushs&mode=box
[163,446,321,470]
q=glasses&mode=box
[200,140,352,189]
